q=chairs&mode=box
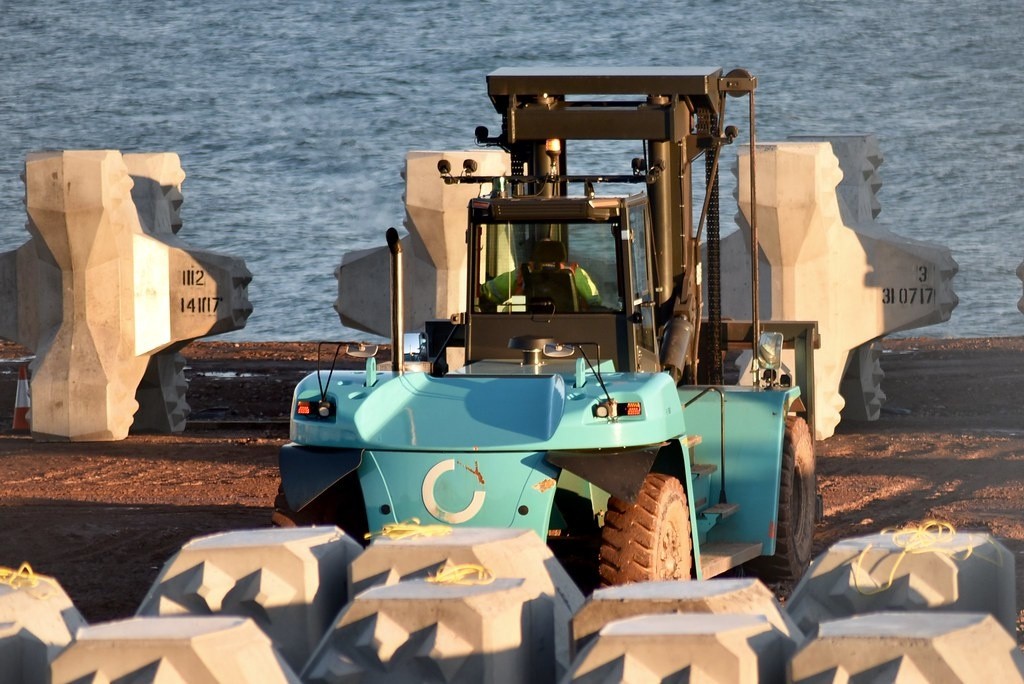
[521,239,580,313]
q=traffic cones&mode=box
[6,364,31,435]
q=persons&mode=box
[479,239,602,312]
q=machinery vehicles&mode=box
[273,61,830,592]
